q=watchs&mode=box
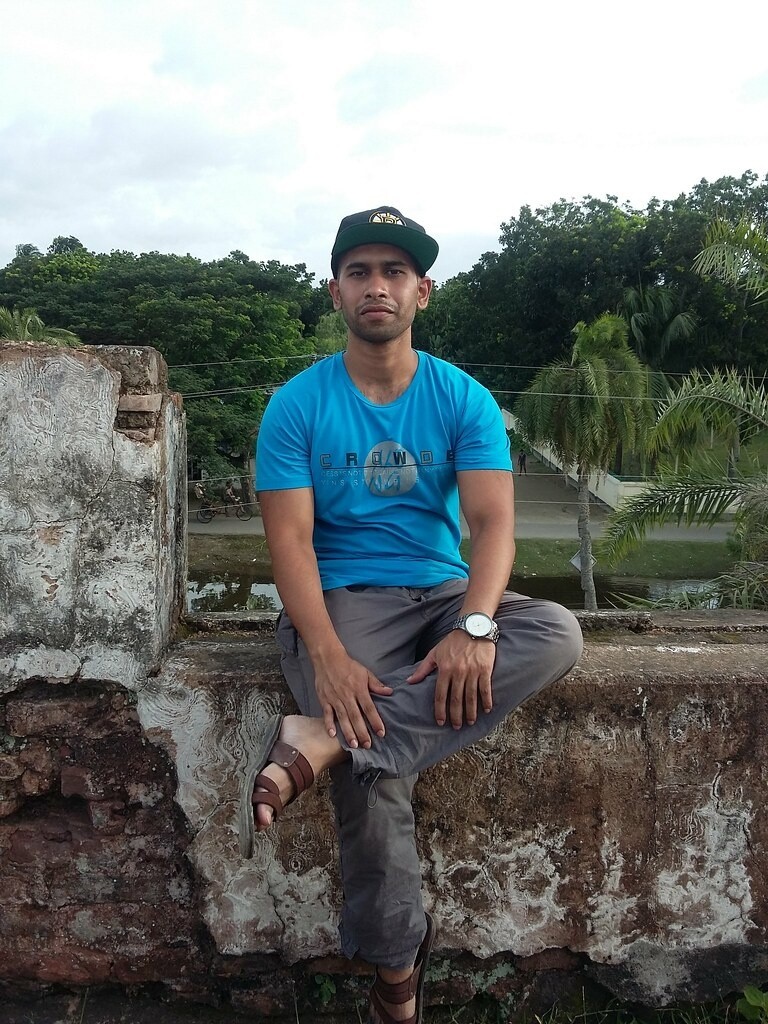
[452,612,500,643]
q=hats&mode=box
[331,206,439,273]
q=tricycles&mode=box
[194,480,253,523]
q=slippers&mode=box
[238,713,316,860]
[369,911,436,1024]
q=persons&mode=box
[236,201,585,1023]
